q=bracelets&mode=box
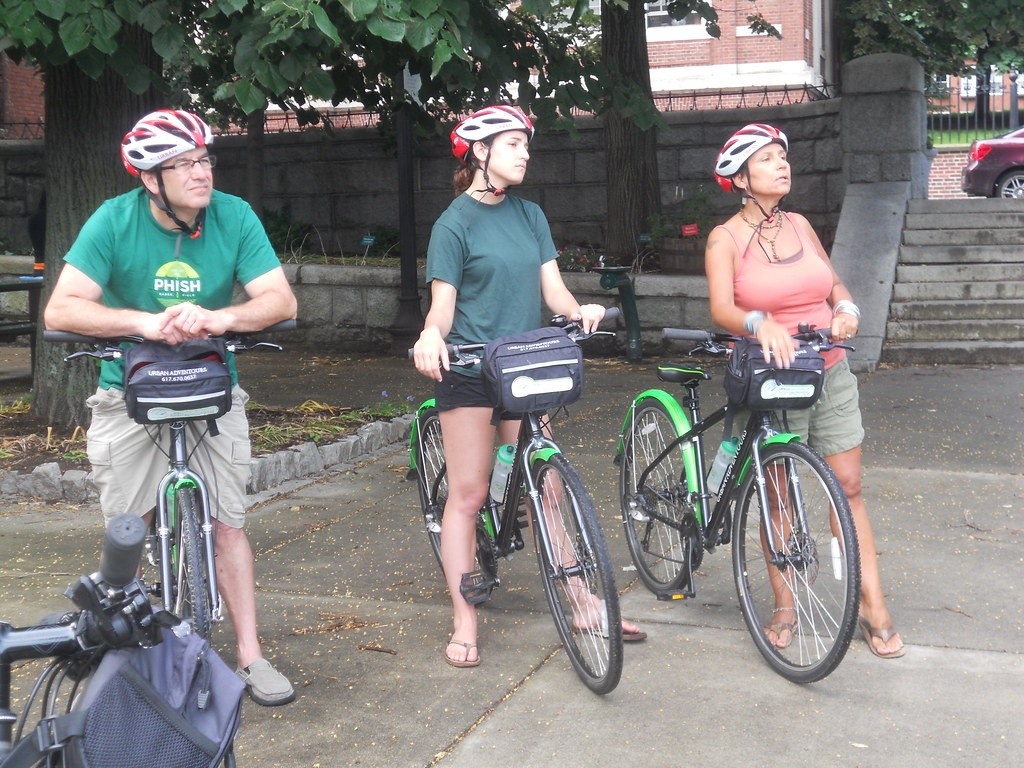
[832,300,861,320]
[743,310,766,336]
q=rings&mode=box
[771,339,776,349]
[846,334,852,339]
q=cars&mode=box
[961,125,1024,199]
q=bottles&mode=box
[167,484,175,534]
[489,443,515,504]
[707,436,739,495]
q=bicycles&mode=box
[407,306,625,694]
[612,321,862,684]
[0,512,247,767]
[45,319,297,650]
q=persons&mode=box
[43,109,297,706]
[412,105,647,667]
[705,124,907,658]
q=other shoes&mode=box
[236,658,295,706]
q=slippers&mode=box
[444,631,481,668]
[857,615,907,658]
[760,616,798,650]
[570,598,647,641]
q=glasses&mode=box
[154,153,217,173]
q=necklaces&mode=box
[737,206,785,263]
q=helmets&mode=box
[714,122,788,193]
[121,109,214,181]
[450,104,535,164]
